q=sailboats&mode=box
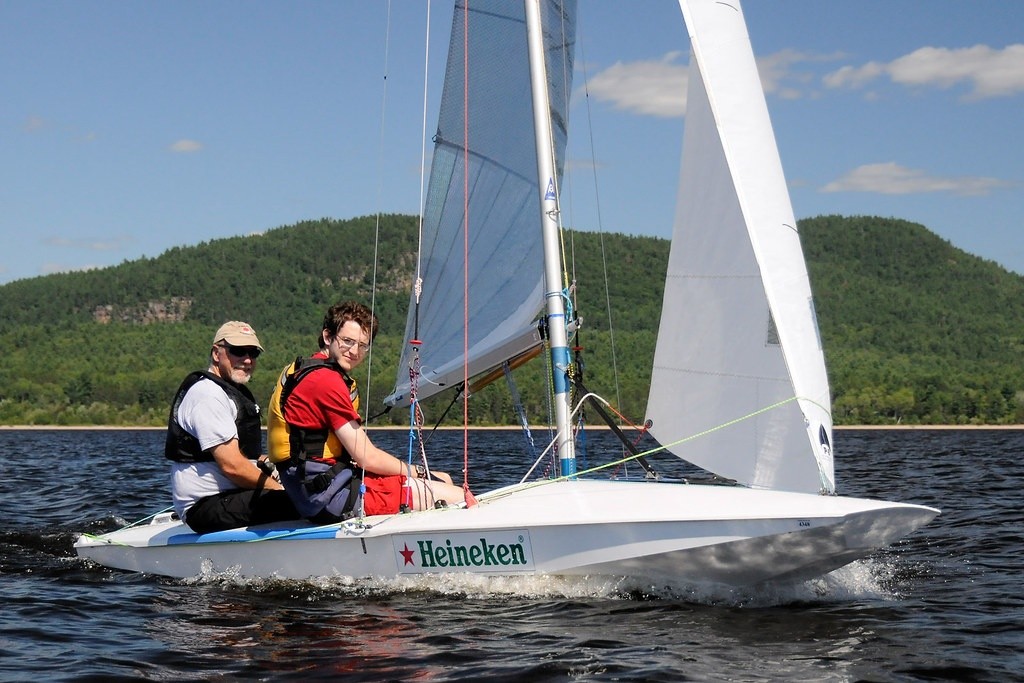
[71,1,942,585]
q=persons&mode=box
[165,321,306,534]
[267,302,465,523]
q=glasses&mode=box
[331,330,370,352]
[211,344,261,358]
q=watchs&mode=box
[416,465,425,478]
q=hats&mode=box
[212,321,265,353]
